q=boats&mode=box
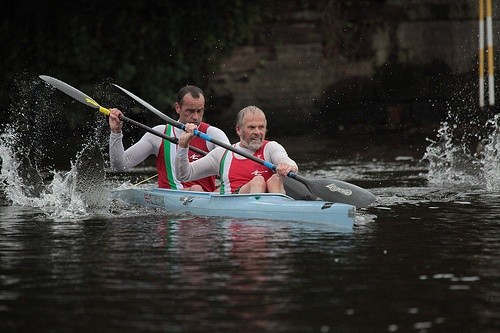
[110,187,356,230]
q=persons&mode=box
[174,106,298,194]
[108,86,231,192]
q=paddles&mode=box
[112,83,376,208]
[38,74,208,155]
